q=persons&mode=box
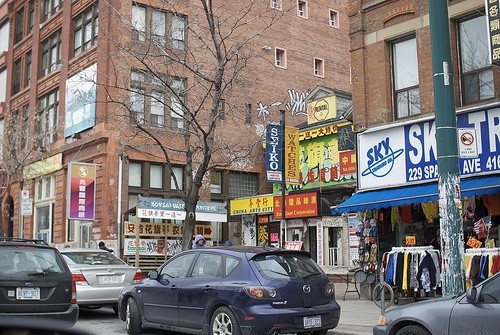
[191,234,210,249]
[98,241,114,254]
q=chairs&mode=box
[202,260,218,277]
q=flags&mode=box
[473,215,492,240]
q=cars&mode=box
[372,269,500,335]
[0,237,79,335]
[34,247,142,316]
[116,243,341,335]
